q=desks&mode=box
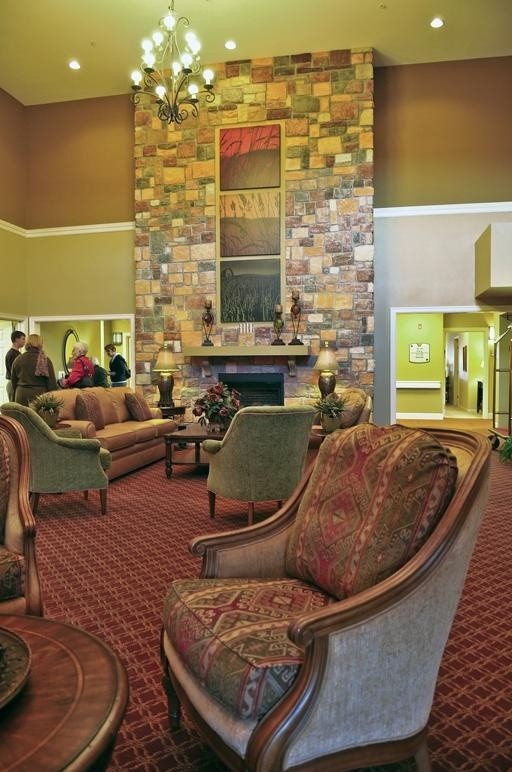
[162,424,227,479]
[159,405,186,429]
[2,613,131,770]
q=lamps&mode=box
[112,330,123,346]
[313,341,340,402]
[151,343,180,405]
[129,2,216,127]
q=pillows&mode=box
[75,392,104,431]
[125,392,153,422]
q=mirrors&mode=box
[62,327,80,375]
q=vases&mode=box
[205,418,226,431]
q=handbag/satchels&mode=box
[71,375,93,388]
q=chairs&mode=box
[202,405,315,526]
[0,412,44,619]
[161,423,490,772]
[1,402,112,515]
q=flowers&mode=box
[192,381,241,434]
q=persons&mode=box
[103,345,132,387]
[59,342,95,388]
[90,358,108,387]
[10,333,58,405]
[4,330,25,402]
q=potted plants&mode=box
[29,390,65,426]
[311,393,345,432]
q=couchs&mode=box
[36,386,177,482]
